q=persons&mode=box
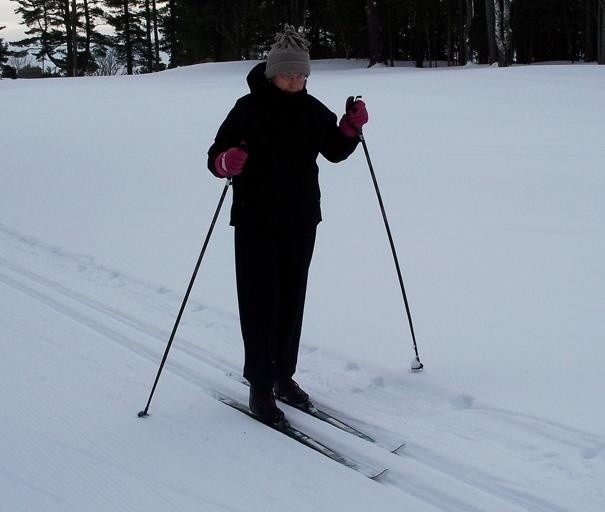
[206,28,367,423]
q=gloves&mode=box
[339,95,368,137]
[214,147,249,177]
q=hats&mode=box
[264,23,311,81]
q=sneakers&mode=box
[249,383,284,423]
[274,377,308,406]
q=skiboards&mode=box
[207,369,408,479]
